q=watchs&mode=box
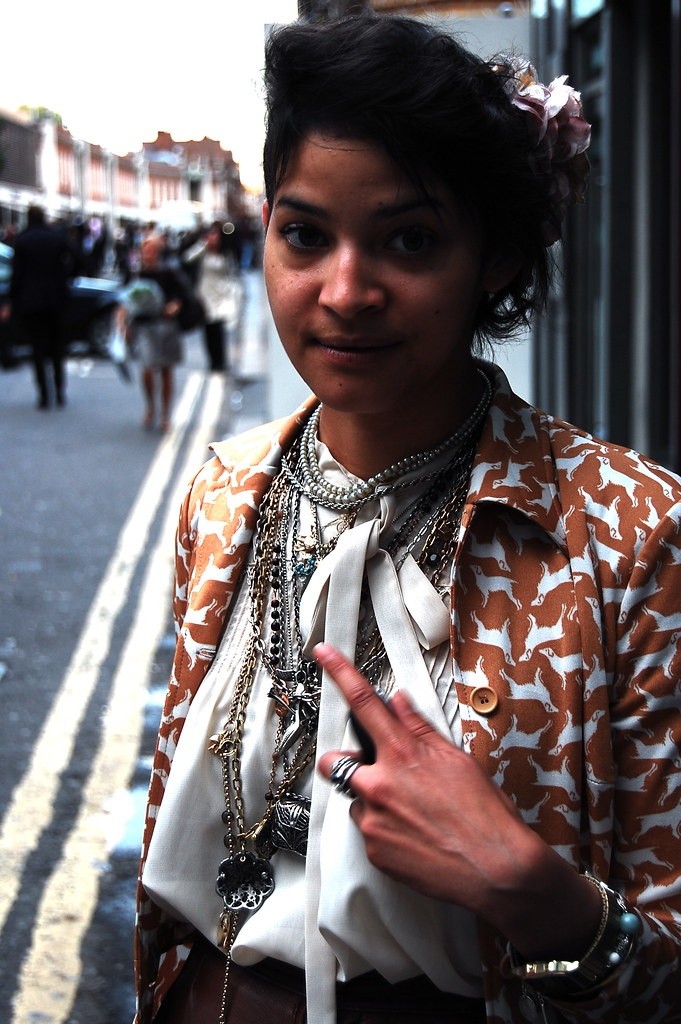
[506,875,629,995]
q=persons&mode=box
[0,207,76,411]
[134,16,681,1024]
[0,202,261,374]
[116,234,189,431]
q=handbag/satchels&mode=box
[110,326,127,363]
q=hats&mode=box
[119,280,164,319]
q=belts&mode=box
[253,953,486,1014]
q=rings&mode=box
[330,756,363,798]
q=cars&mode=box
[0,243,127,358]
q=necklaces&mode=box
[195,367,496,1024]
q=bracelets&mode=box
[542,908,640,1002]
